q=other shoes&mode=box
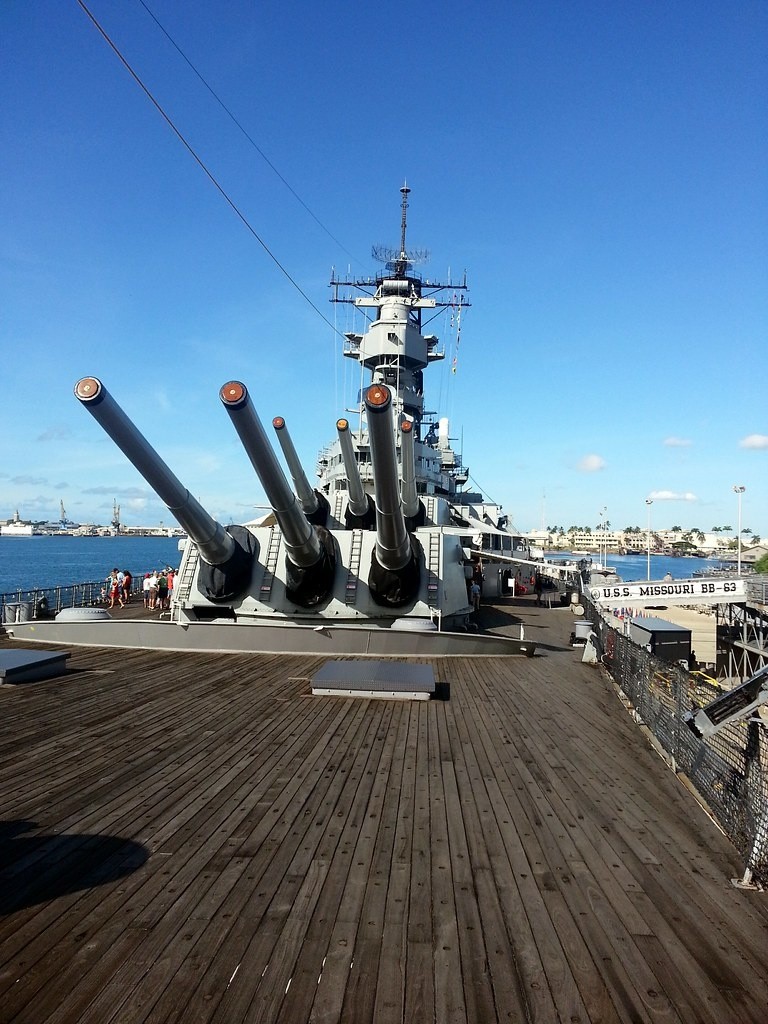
[108,606,114,609]
[148,606,156,612]
[128,601,131,604]
[123,601,127,604]
[120,605,124,609]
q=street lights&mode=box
[597,503,608,571]
[733,484,746,578]
[644,496,655,584]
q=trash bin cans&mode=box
[574,622,595,643]
[1,601,34,623]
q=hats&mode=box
[113,568,118,571]
[145,573,150,578]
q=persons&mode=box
[688,650,697,671]
[470,580,481,611]
[662,572,672,581]
[100,568,179,610]
[533,578,543,602]
